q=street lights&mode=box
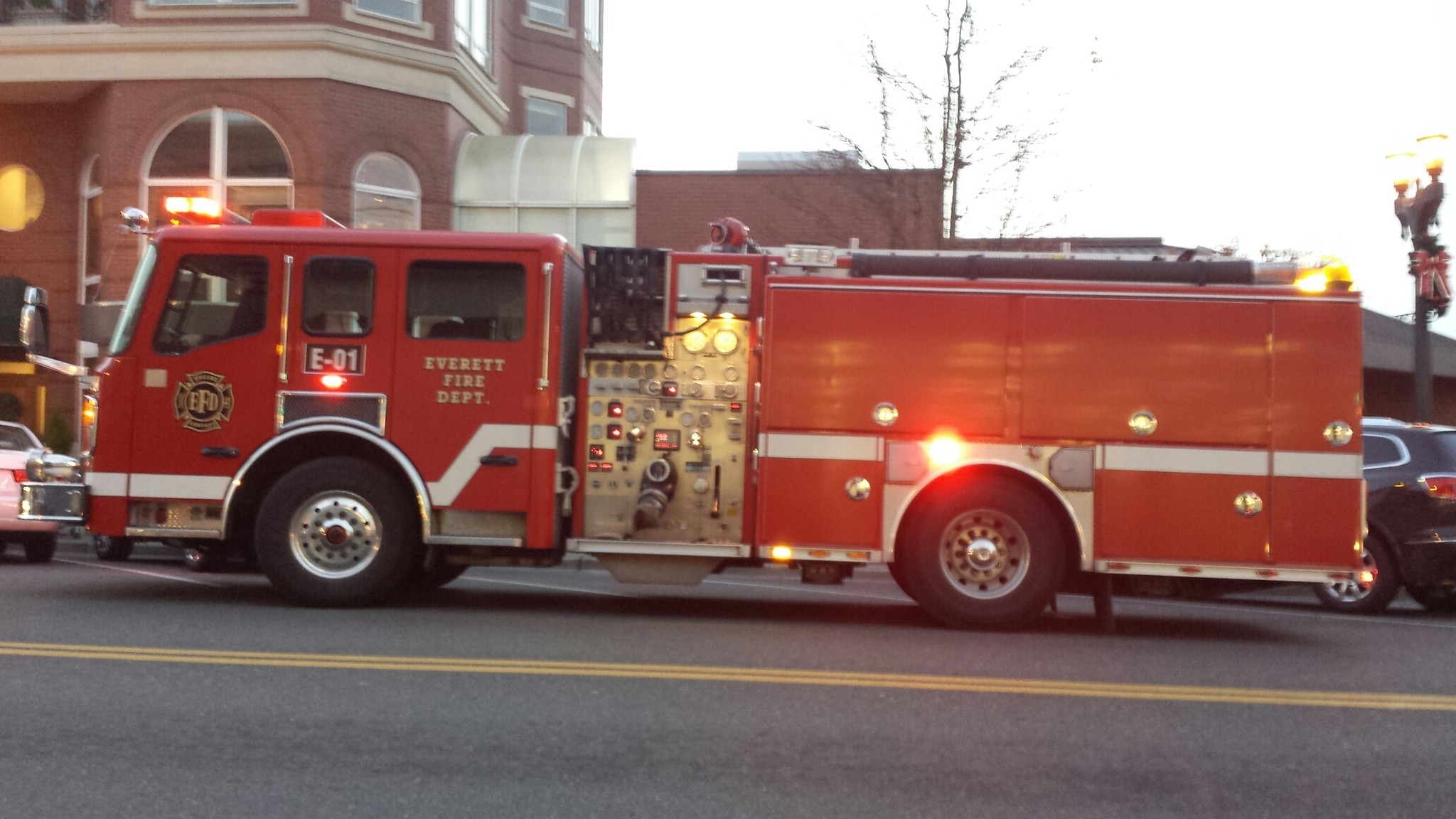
[1381,124,1455,425]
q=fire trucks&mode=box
[20,193,1374,635]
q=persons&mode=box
[179,263,265,348]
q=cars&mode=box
[0,420,63,564]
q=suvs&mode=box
[1312,415,1456,618]
